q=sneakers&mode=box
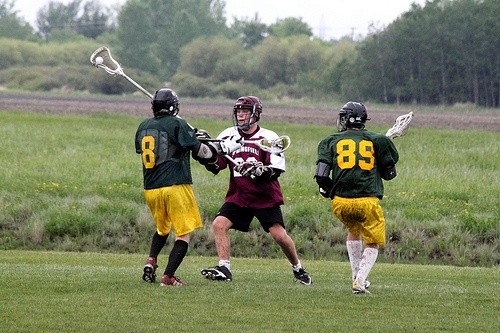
[160,274,187,286]
[200,266,233,281]
[293,266,313,285]
[353,281,370,288]
[142,256,158,282]
[353,285,370,294]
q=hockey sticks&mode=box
[194,135,291,155]
[318,112,414,197]
[89,45,260,183]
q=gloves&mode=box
[196,129,211,138]
[221,135,245,154]
[233,161,263,177]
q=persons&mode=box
[134,88,219,288]
[193,95,312,286]
[313,101,399,294]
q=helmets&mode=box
[336,101,368,132]
[152,88,180,117]
[231,96,263,131]
